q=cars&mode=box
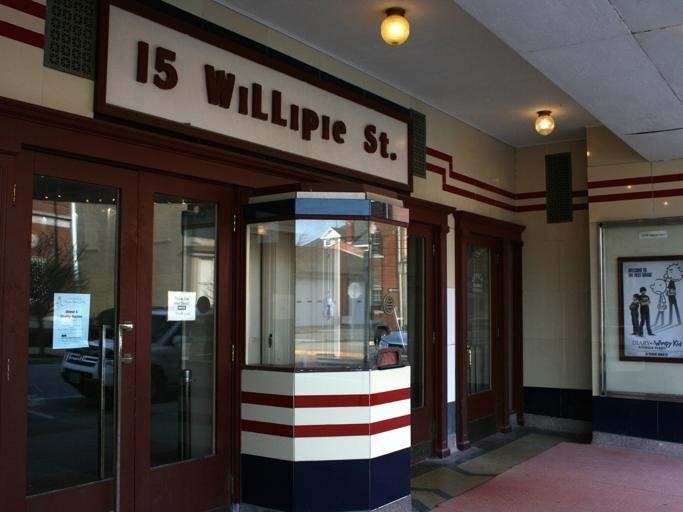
[59,305,215,404]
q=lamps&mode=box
[380,6,409,51]
[533,109,554,139]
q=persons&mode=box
[628,293,638,334]
[638,287,654,337]
